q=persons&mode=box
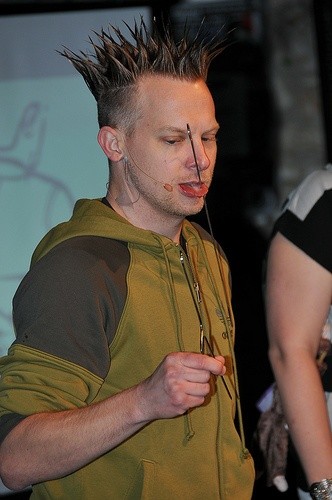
[0,8,255,500]
[266,163,332,500]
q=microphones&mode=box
[122,135,173,193]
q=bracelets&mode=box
[310,478,332,500]
